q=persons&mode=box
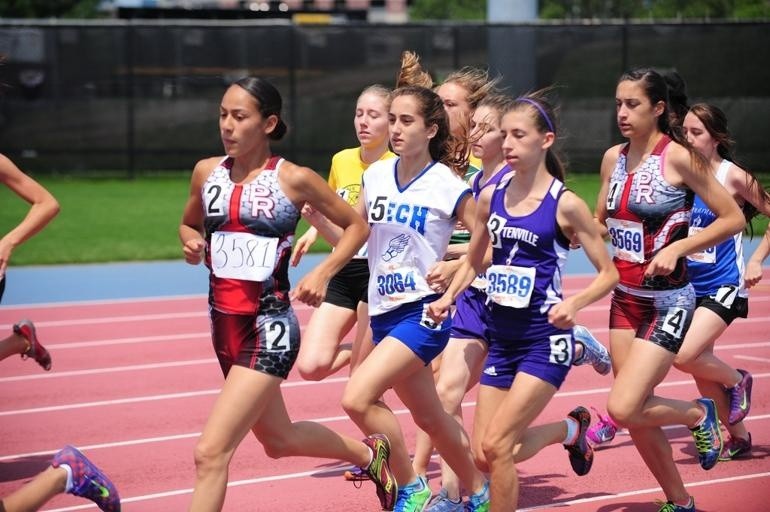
[289,52,621,512]
[0,149,61,372]
[570,65,770,512]
[1,444,125,512]
[171,71,399,510]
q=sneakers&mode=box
[654,495,695,512]
[690,398,723,470]
[345,464,369,480]
[13,320,52,371]
[564,406,594,476]
[719,432,752,461]
[728,369,752,425]
[586,406,618,445]
[391,474,491,512]
[48,445,120,512]
[573,324,611,376]
[354,433,398,511]
